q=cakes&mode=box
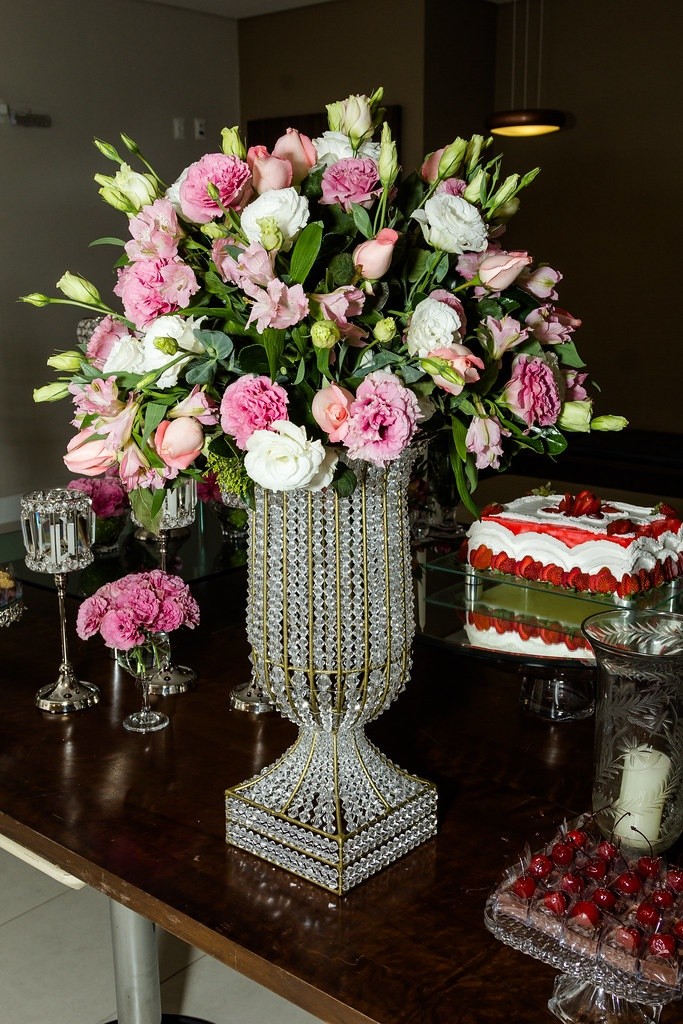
[463,603,666,661]
[460,482,683,599]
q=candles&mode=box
[613,748,668,848]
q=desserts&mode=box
[497,804,683,987]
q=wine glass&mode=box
[115,474,197,695]
[20,490,101,713]
[115,630,171,731]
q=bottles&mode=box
[581,607,683,855]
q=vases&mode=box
[226,435,438,895]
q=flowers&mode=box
[16,87,630,535]
[69,463,222,540]
[77,569,200,671]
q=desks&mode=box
[0,523,683,1024]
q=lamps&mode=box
[486,0,565,137]
